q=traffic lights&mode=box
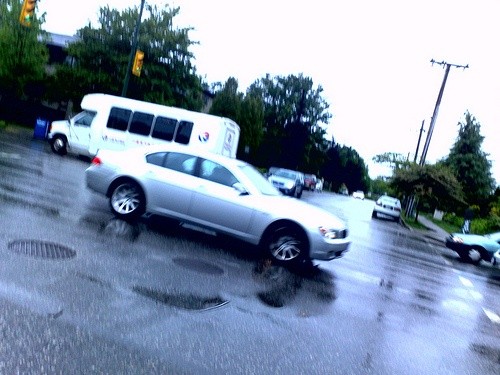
[19,0,37,26]
[132,48,145,78]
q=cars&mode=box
[302,174,317,192]
[353,190,365,200]
[316,178,323,192]
[267,168,304,198]
[86,145,351,270]
[444,231,500,264]
[338,187,349,195]
[371,195,402,222]
[491,249,500,269]
[263,167,279,180]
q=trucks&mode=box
[48,92,242,163]
